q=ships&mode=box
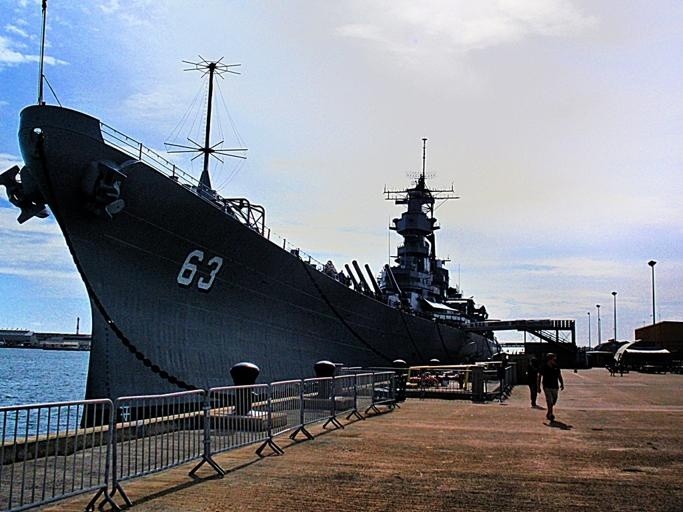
[0,0,504,426]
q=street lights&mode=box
[586,310,591,348]
[595,303,601,346]
[610,290,617,341]
[647,260,657,344]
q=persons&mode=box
[618,357,625,377]
[322,260,364,291]
[526,357,540,406]
[537,352,564,420]
